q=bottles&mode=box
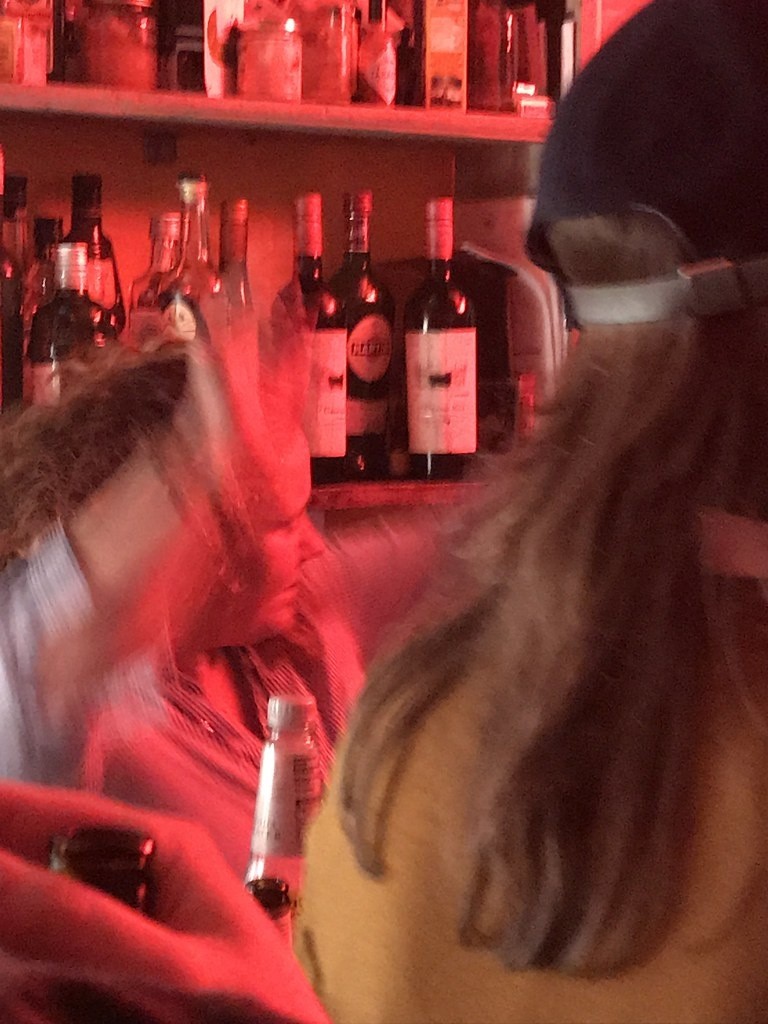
[470,0,515,113]
[32,824,154,1024]
[246,696,325,902]
[405,199,478,479]
[358,0,396,107]
[0,170,124,413]
[243,877,294,952]
[267,189,395,482]
[232,18,303,102]
[0,0,204,93]
[394,26,422,107]
[127,174,262,416]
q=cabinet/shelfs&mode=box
[0,0,657,517]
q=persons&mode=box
[302,1,767,1024]
[1,784,323,1024]
[0,273,364,941]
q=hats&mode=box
[522,0,768,324]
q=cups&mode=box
[311,0,353,104]
